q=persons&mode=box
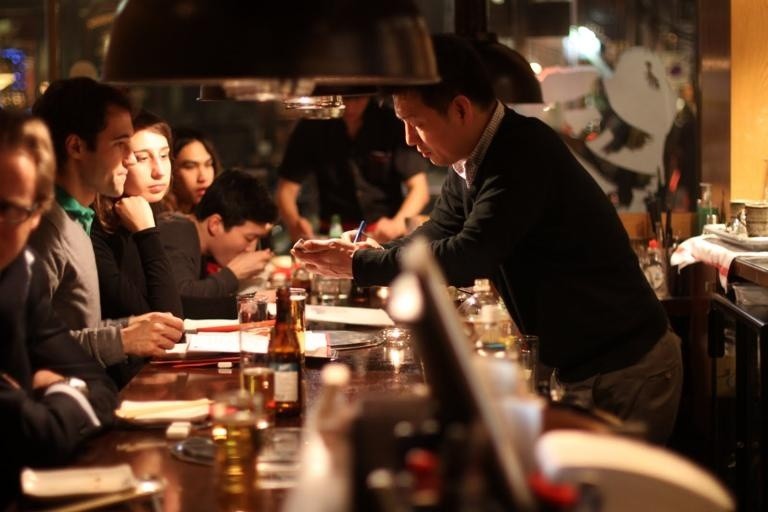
[154,132,218,214]
[29,74,186,373]
[155,167,277,317]
[292,28,685,448]
[89,107,189,322]
[0,102,124,502]
[275,92,432,244]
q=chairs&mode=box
[702,294,765,499]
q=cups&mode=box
[207,284,309,496]
[728,201,768,238]
[509,335,540,399]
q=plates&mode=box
[113,396,211,428]
[19,464,140,500]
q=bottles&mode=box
[328,214,343,239]
[642,246,669,301]
[455,278,500,345]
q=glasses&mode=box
[0,199,39,227]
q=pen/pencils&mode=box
[645,198,672,247]
[354,220,365,243]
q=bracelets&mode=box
[44,377,89,397]
